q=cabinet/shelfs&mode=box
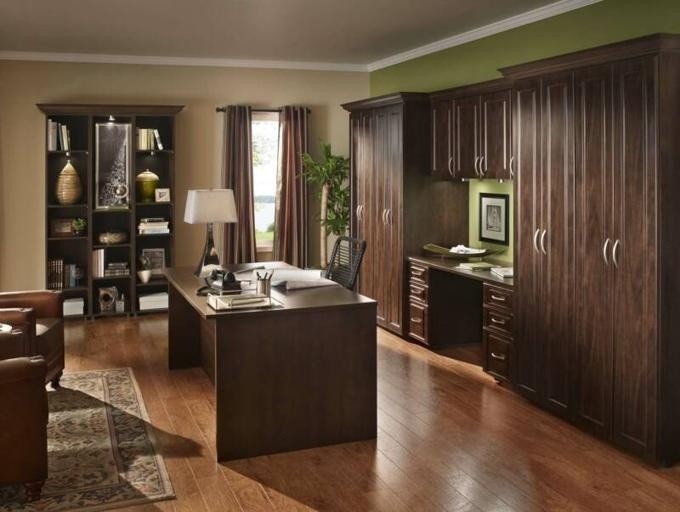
[428,76,518,180]
[497,53,584,428]
[339,33,680,470]
[36,104,187,319]
[340,90,469,338]
[405,255,524,391]
[577,35,680,469]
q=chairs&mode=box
[0,290,65,502]
[325,236,366,291]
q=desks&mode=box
[160,261,378,462]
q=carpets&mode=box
[0,367,175,512]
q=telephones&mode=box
[207,269,242,290]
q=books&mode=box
[133,123,165,150]
[458,260,500,272]
[48,118,72,152]
[491,266,513,278]
[46,255,87,289]
[92,247,130,279]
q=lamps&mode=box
[183,188,239,278]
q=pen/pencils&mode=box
[257,269,274,281]
